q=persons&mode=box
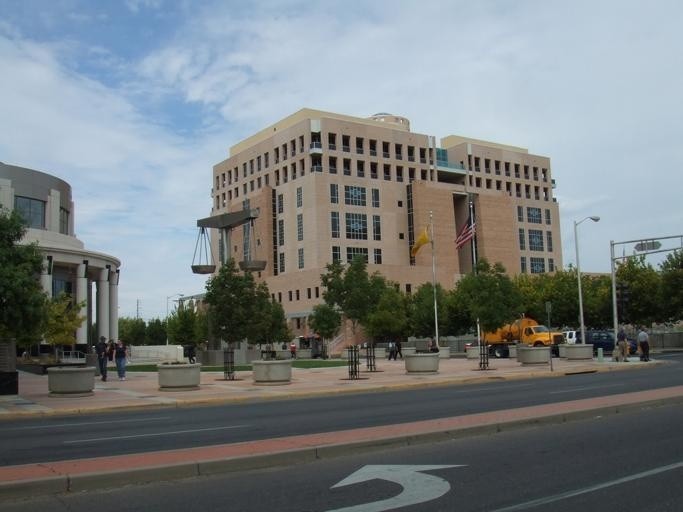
[427,335,439,354]
[394,336,403,361]
[388,340,396,362]
[617,325,651,362]
[95,337,128,382]
[290,342,309,360]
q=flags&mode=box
[454,216,478,247]
[410,225,435,255]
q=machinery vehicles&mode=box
[480,316,564,359]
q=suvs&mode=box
[577,330,637,355]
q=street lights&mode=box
[165,293,184,345]
[573,216,601,343]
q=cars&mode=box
[464,339,491,353]
[561,331,576,345]
